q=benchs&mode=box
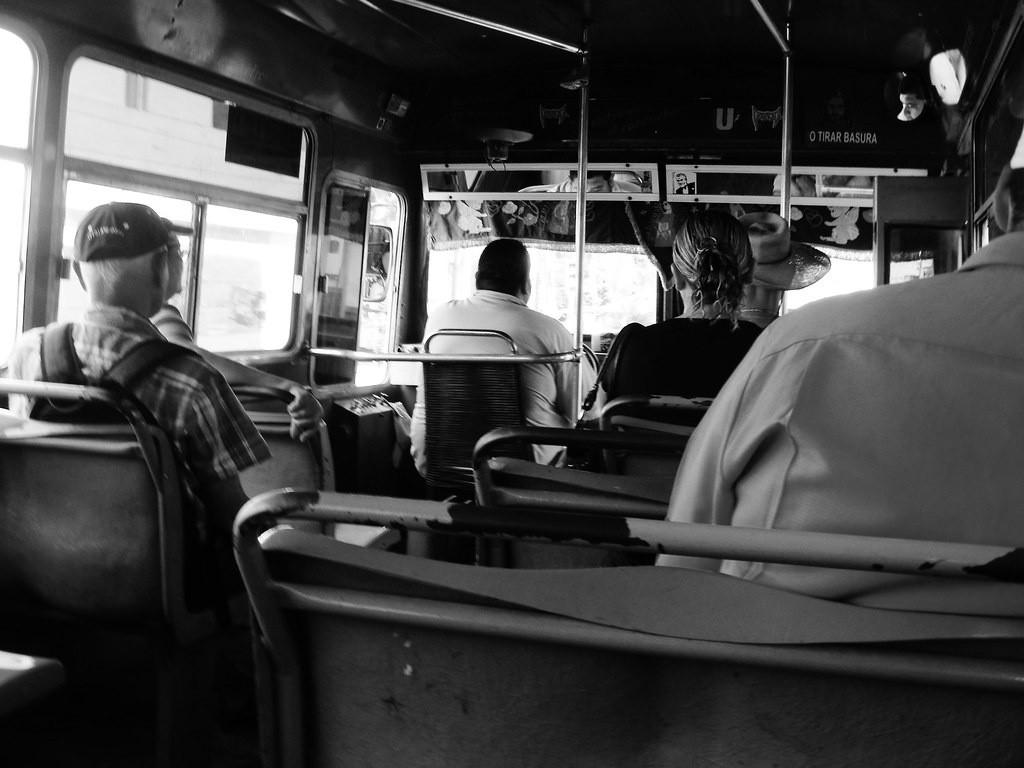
[231,392,1024,768]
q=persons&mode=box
[7,200,269,606]
[512,172,647,193]
[676,173,694,195]
[156,213,323,441]
[408,237,575,481]
[733,207,832,329]
[770,173,873,198]
[657,166,1024,616]
[603,212,766,402]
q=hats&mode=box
[736,210,829,290]
[74,203,167,261]
[161,215,194,236]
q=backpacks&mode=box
[31,324,205,562]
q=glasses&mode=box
[170,250,188,261]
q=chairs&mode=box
[0,326,533,767]
